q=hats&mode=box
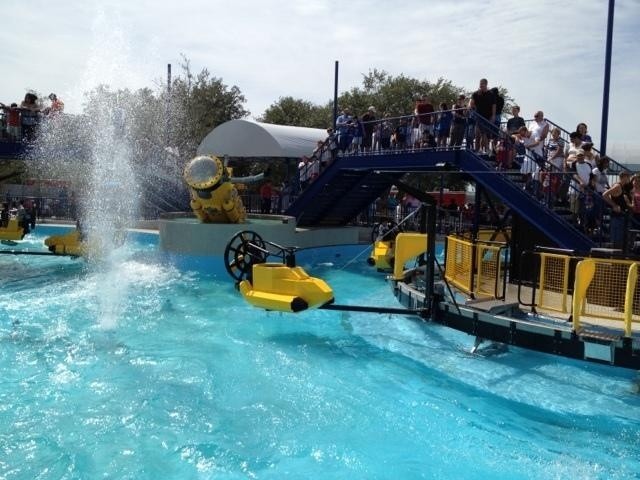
[341,108,352,116]
[368,106,377,113]
[576,149,585,156]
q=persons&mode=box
[6,93,64,143]
[0,185,75,227]
